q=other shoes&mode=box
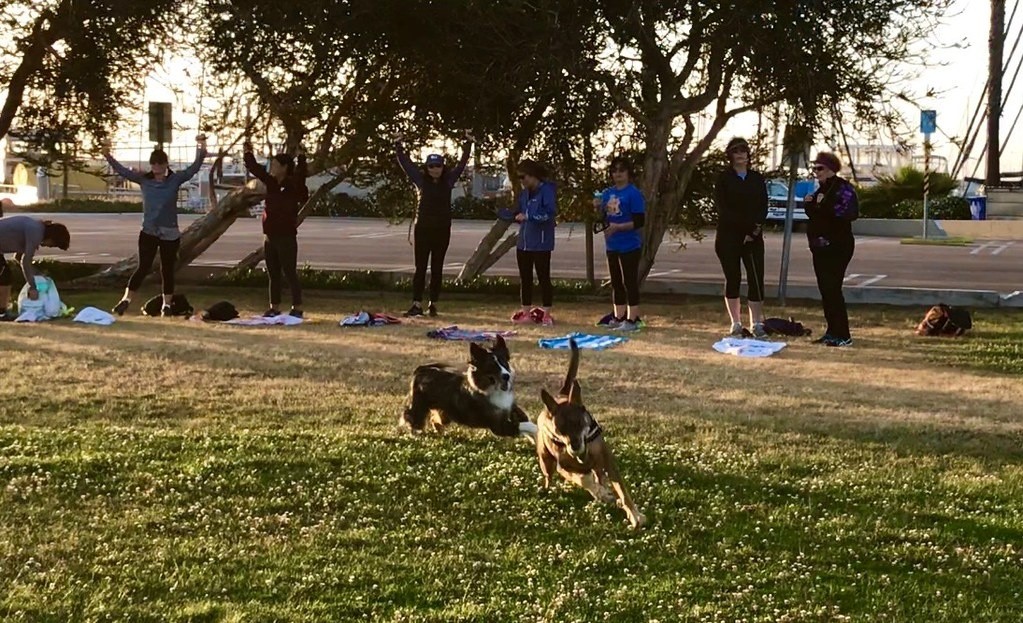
[512,312,535,324]
[541,317,553,328]
[112,298,131,317]
[161,306,173,320]
[427,305,437,318]
[289,309,304,319]
[403,305,424,318]
[0,310,20,322]
[262,309,281,318]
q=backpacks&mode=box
[917,304,971,336]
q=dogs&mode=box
[400,334,647,533]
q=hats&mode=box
[426,154,442,164]
[813,151,842,173]
[725,136,749,151]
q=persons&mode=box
[242,140,308,318]
[803,150,861,348]
[496,158,558,328]
[100,133,208,317]
[392,131,474,317]
[595,155,647,331]
[711,137,770,339]
[0,215,70,321]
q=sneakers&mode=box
[730,323,742,337]
[749,321,768,339]
[812,334,852,348]
[603,319,641,333]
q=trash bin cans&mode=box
[967,195,987,220]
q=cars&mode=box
[684,182,810,232]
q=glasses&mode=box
[811,166,824,172]
[518,174,527,180]
[429,164,441,168]
[733,146,747,153]
[611,166,626,173]
